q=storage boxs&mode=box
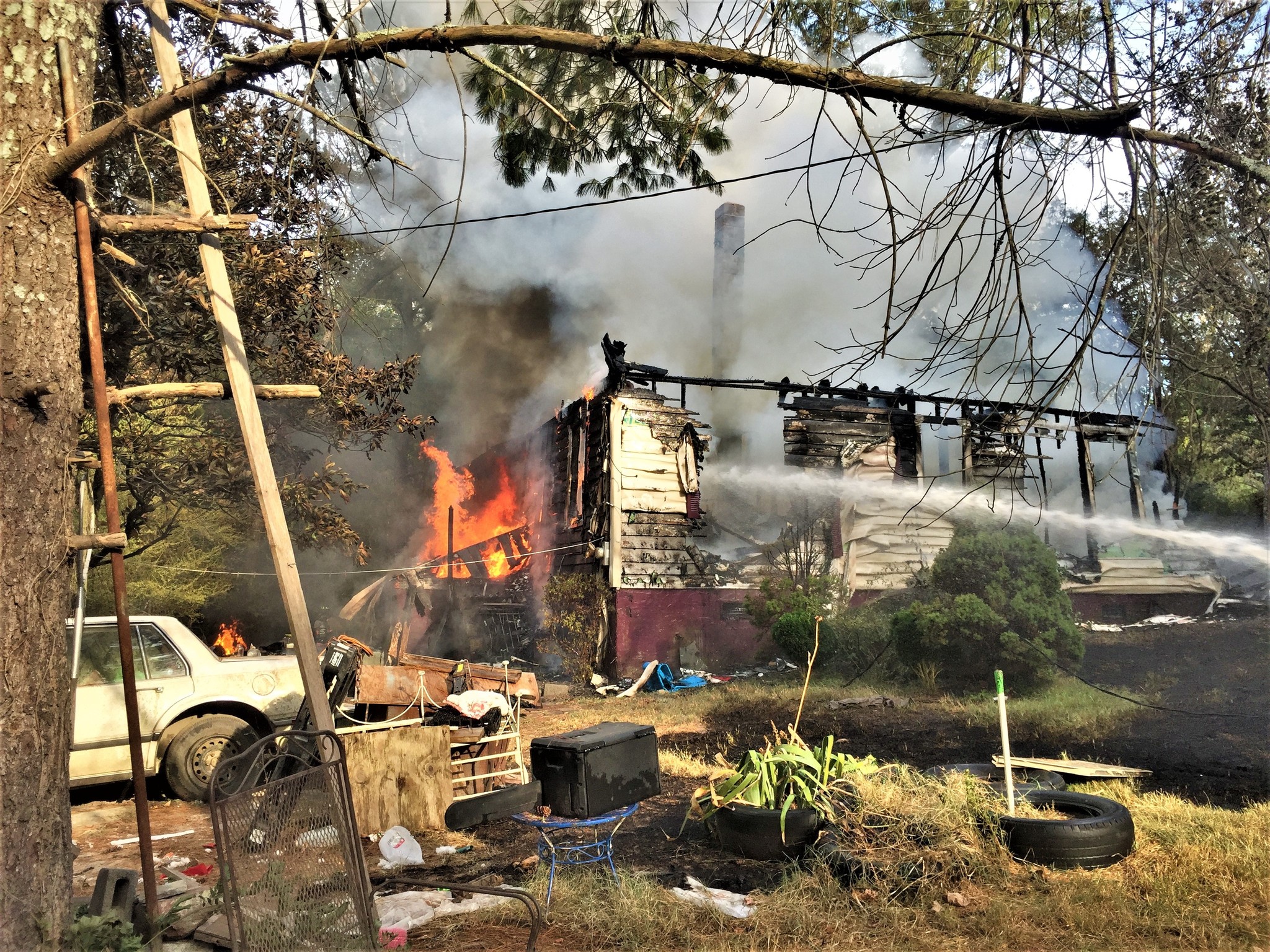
[530,720,661,820]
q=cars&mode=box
[66,614,337,804]
